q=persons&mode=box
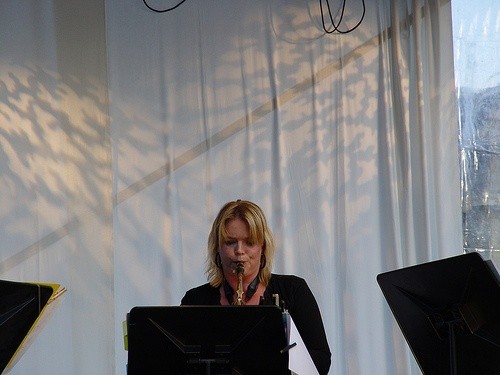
[179,199,331,375]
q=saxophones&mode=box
[232,261,245,305]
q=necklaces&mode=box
[222,273,261,305]
